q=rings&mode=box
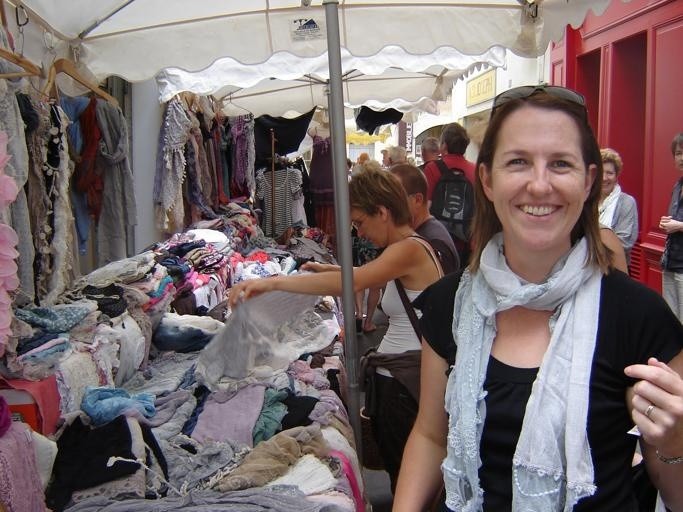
[644,402,656,419]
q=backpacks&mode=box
[428,157,476,246]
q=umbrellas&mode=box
[0,0,609,466]
[163,39,516,255]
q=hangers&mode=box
[1,23,120,108]
[262,157,303,172]
[168,90,253,119]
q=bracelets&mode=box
[654,451,682,465]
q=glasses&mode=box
[490,85,589,125]
[351,209,370,230]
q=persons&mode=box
[392,85,683,512]
[355,163,385,334]
[595,148,638,276]
[226,170,448,512]
[304,119,336,207]
[343,145,407,165]
[416,136,440,169]
[420,122,481,267]
[658,133,683,327]
[392,164,461,278]
[594,220,628,275]
